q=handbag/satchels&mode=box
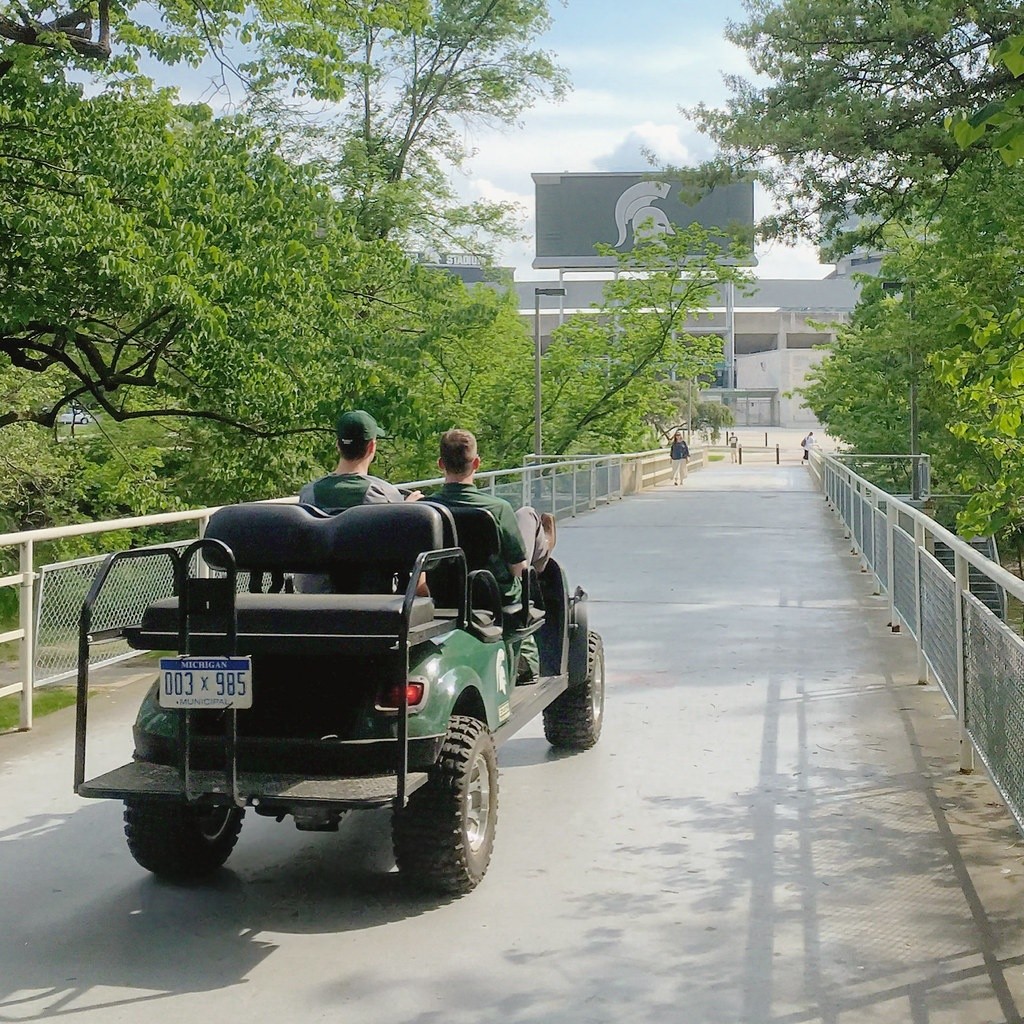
[801,439,806,446]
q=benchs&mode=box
[140,506,444,634]
[249,502,495,621]
[321,507,535,630]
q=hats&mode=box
[337,410,385,441]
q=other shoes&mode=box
[530,512,556,572]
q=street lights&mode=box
[883,281,922,501]
[687,362,704,445]
[533,286,568,501]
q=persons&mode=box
[669,433,690,484]
[801,431,814,464]
[293,410,425,597]
[417,430,556,596]
[729,432,739,463]
